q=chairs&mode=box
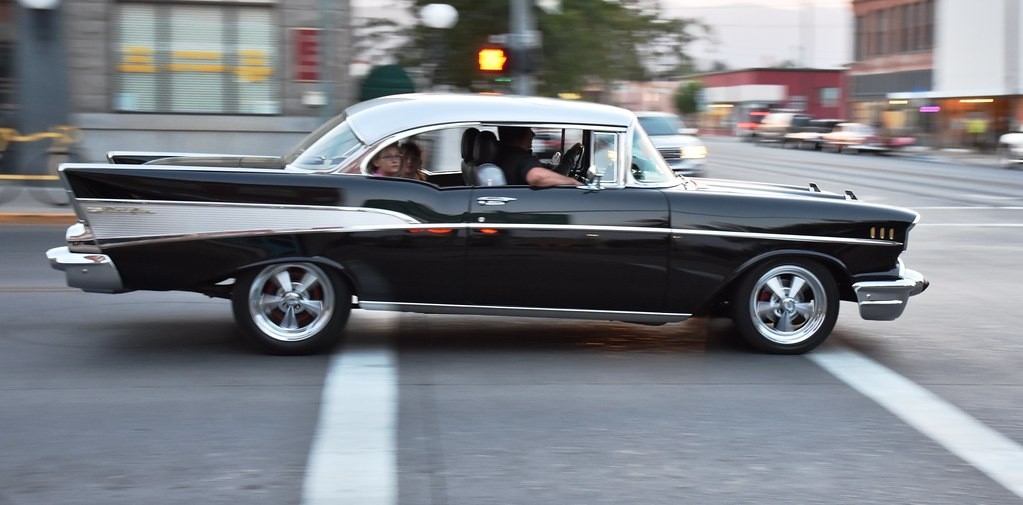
[461,128,479,186]
[472,131,506,187]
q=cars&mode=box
[44,92,928,357]
[611,111,708,176]
[730,106,900,155]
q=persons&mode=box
[369,140,427,181]
[495,125,584,188]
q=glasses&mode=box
[375,153,405,161]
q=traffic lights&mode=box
[467,48,516,84]
